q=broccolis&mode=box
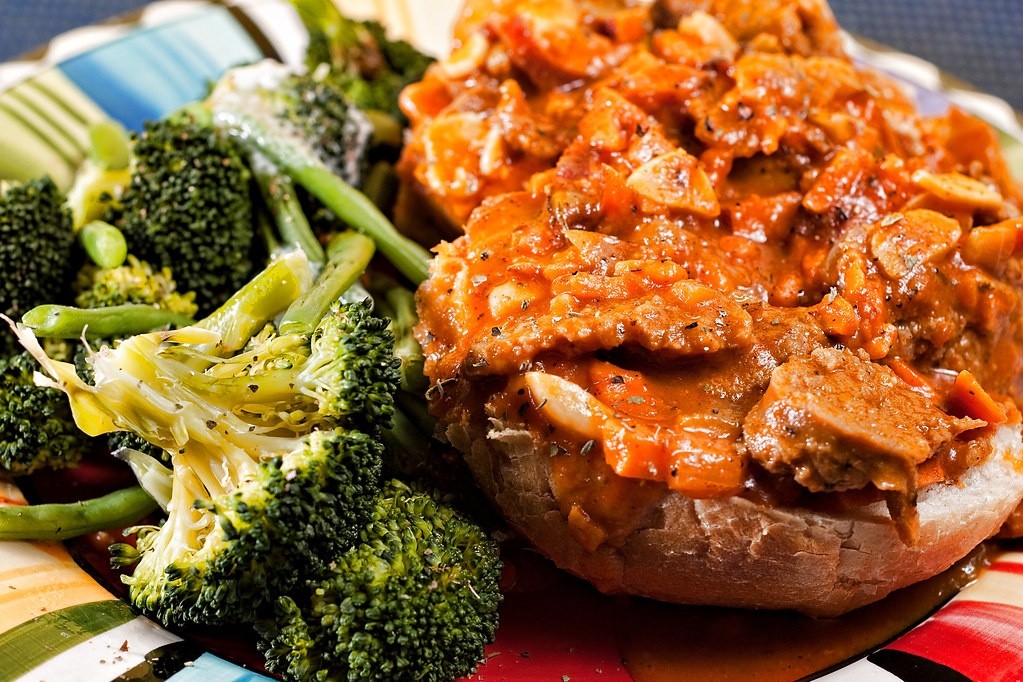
[1,1,503,682]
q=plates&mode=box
[0,0,1023,682]
